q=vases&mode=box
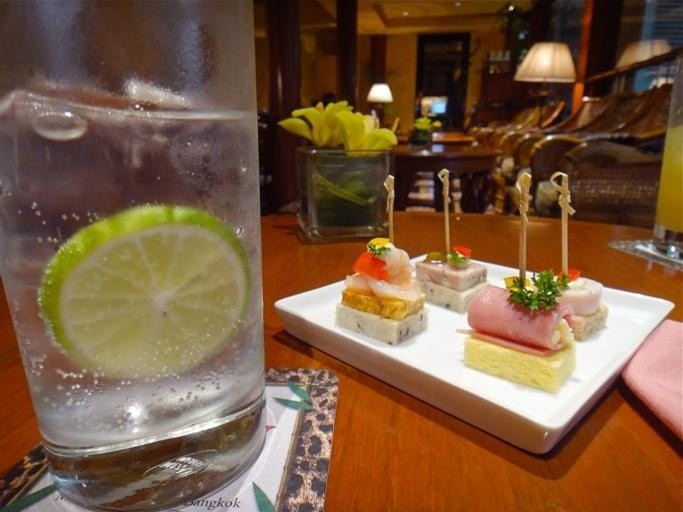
[295,143,390,248]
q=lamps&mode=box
[513,42,578,130]
[365,82,394,128]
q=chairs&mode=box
[474,83,675,219]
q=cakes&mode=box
[411,249,488,312]
[337,239,433,345]
[547,270,608,341]
[465,284,578,391]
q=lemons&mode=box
[41,205,255,379]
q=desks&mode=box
[392,140,497,216]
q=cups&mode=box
[652,55,683,260]
[0,0,269,512]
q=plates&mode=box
[274,251,676,455]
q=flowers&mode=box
[278,100,397,154]
